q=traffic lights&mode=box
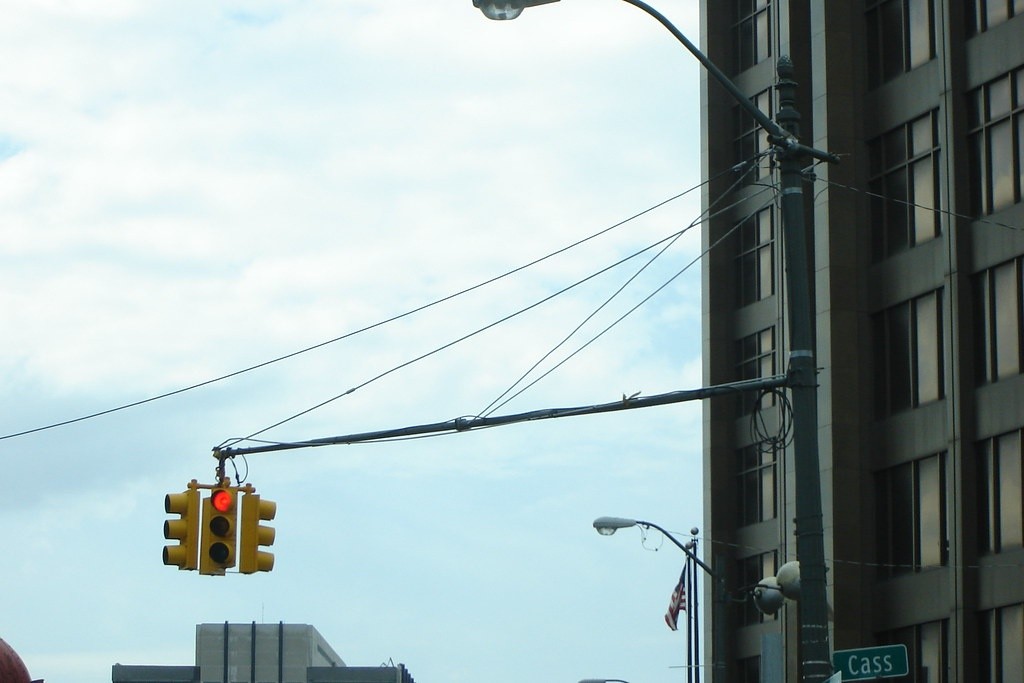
[207,484,238,568]
[163,489,201,571]
[240,493,277,574]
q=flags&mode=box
[664,563,687,632]
